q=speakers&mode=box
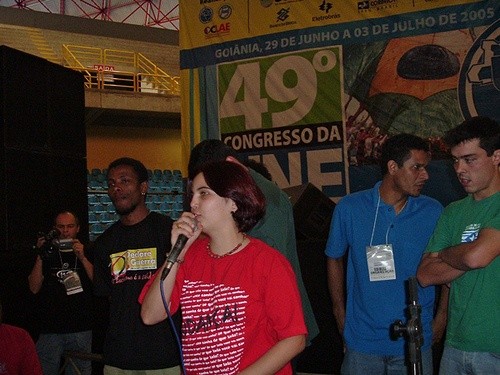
[281,183,337,242]
[4,44,86,361]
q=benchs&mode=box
[0,23,179,80]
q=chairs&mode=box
[87,168,184,239]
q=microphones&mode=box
[161,218,197,282]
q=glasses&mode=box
[55,223,78,229]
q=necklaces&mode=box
[206,231,247,259]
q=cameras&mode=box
[44,230,73,254]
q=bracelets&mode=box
[165,252,185,263]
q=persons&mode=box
[188,140,319,348]
[91,157,183,375]
[140,159,308,375]
[28,209,94,375]
[324,133,450,375]
[416,117,500,375]
